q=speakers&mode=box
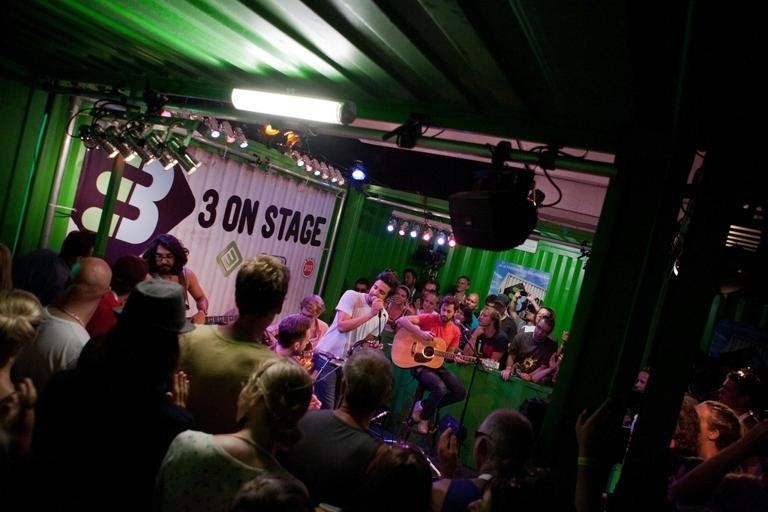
[448,178,539,251]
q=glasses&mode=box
[475,429,492,440]
[240,375,250,387]
[155,254,175,261]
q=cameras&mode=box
[521,291,527,296]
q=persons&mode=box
[592,358,768,511]
[0,230,609,512]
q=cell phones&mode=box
[439,415,466,439]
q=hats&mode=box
[112,278,196,334]
[494,294,509,307]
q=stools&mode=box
[400,369,438,453]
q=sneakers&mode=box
[418,420,429,434]
[412,400,424,422]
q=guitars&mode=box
[391,326,501,370]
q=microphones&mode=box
[370,410,390,422]
[372,296,382,310]
[455,319,470,333]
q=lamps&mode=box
[78,124,202,176]
[291,151,346,186]
[231,86,356,124]
[205,114,248,149]
[385,218,457,248]
